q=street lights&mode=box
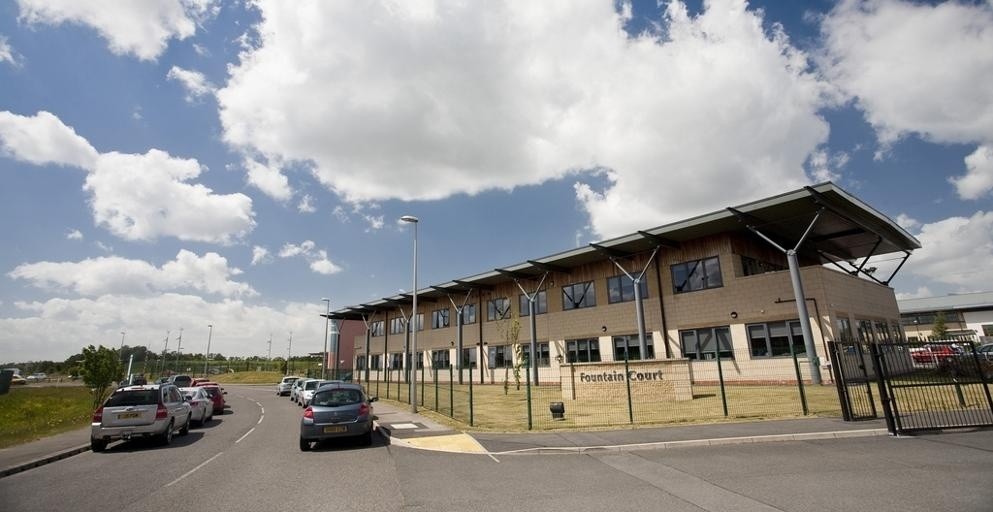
[119,331,126,358]
[401,215,419,414]
[321,298,331,379]
[203,324,213,377]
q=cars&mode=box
[10,372,48,385]
[276,375,379,452]
[911,341,993,367]
[91,373,229,453]
